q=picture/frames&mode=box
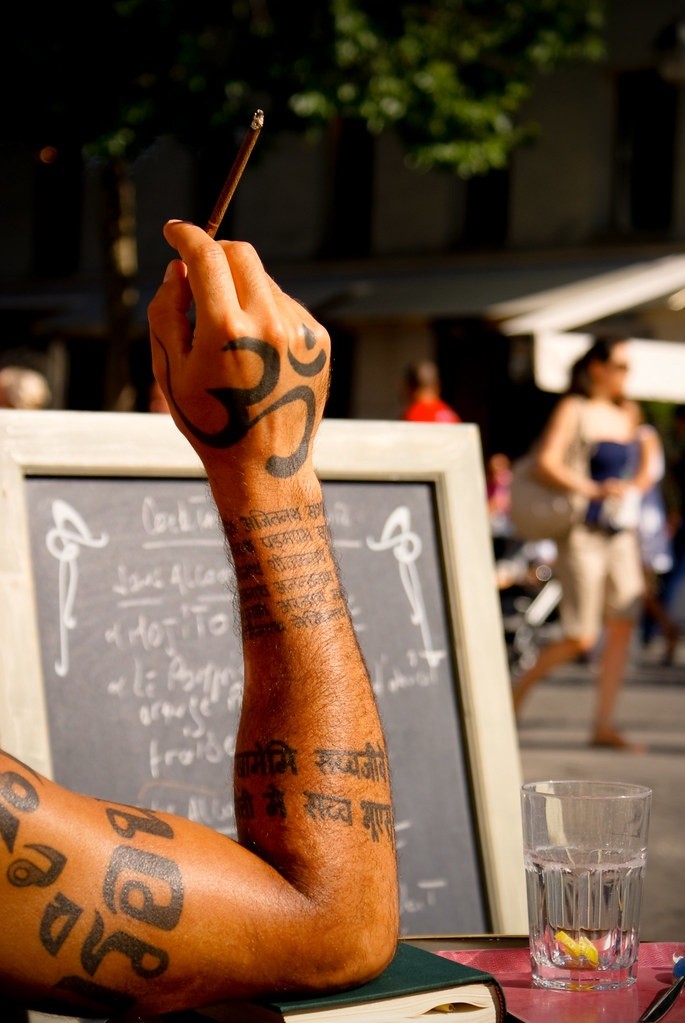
[0,403,533,944]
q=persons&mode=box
[0,365,52,410]
[0,220,399,1012]
[401,359,458,423]
[489,331,684,748]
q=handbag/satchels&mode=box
[511,397,588,538]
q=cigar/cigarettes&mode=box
[205,108,269,242]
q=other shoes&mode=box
[589,734,643,753]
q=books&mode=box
[199,944,507,1023]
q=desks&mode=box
[433,942,685,1023]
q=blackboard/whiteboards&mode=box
[0,408,535,944]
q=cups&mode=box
[519,779,653,990]
